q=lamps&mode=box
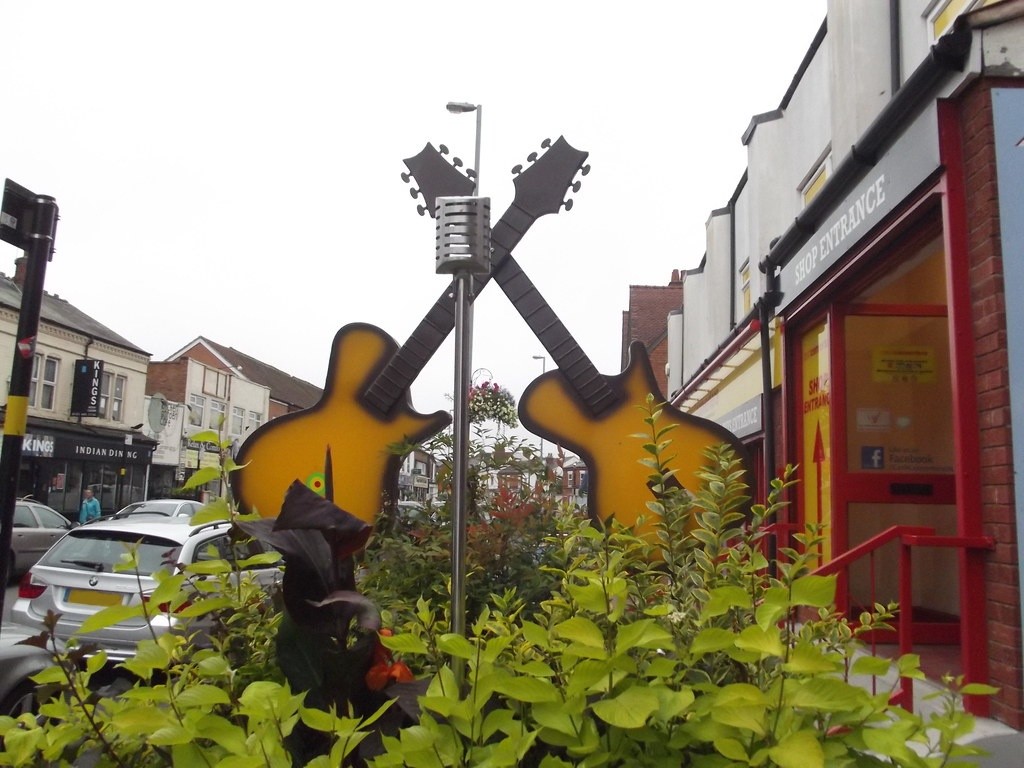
[217,365,243,371]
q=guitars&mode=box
[230,132,756,563]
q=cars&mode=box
[10,511,287,661]
[6,498,82,587]
[0,620,59,756]
[398,497,451,531]
[107,498,209,522]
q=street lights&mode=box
[533,355,546,460]
[446,98,485,198]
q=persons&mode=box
[79,489,101,525]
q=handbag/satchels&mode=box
[86,514,94,523]
